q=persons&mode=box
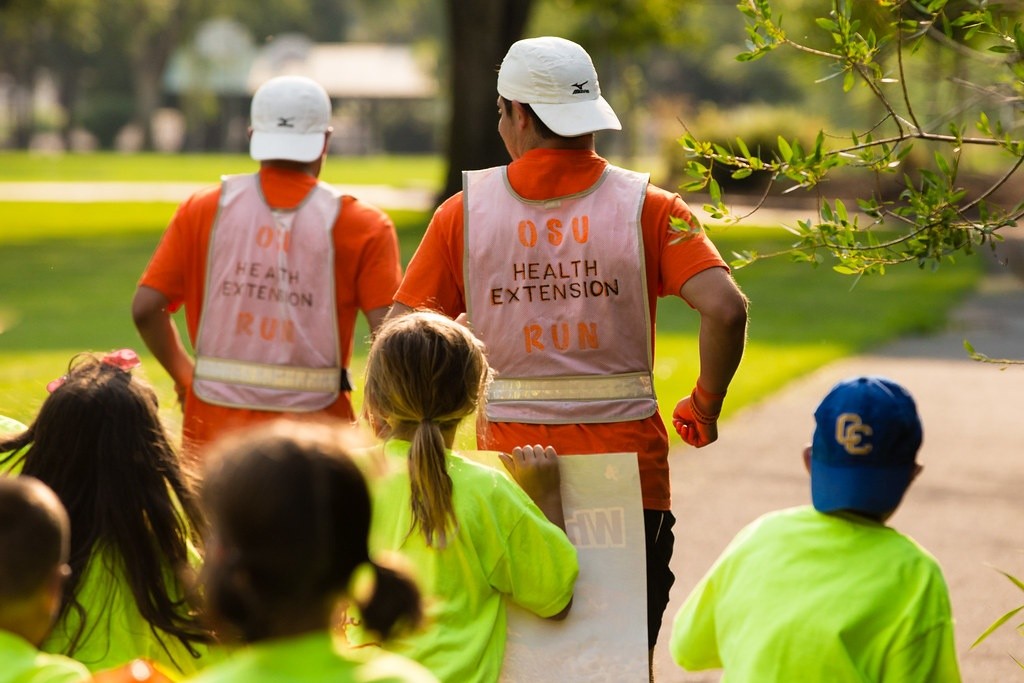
[170,425,438,683]
[344,309,579,682]
[373,37,749,683]
[0,347,217,683]
[133,74,399,476]
[667,377,961,683]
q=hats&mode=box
[250,75,332,163]
[496,36,623,137]
[810,374,924,514]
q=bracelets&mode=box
[689,388,721,427]
[697,379,727,400]
